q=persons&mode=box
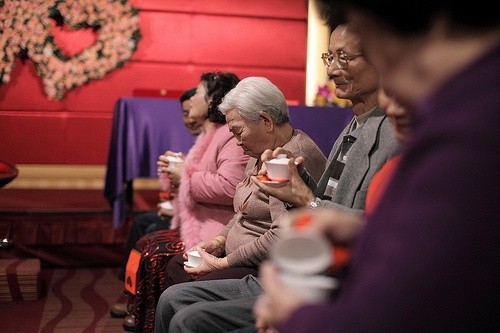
[155,26,408,333]
[254,0,500,333]
[165,77,327,289]
[110,71,250,333]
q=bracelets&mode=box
[212,239,224,250]
[310,197,320,210]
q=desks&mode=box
[103,96,354,231]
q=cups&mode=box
[187,251,201,267]
[266,153,298,181]
[280,273,337,305]
[166,155,183,172]
[159,201,173,214]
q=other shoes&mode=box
[111,303,129,316]
[123,313,137,328]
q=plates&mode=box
[261,180,289,188]
[183,261,194,267]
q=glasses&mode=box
[321,50,363,69]
